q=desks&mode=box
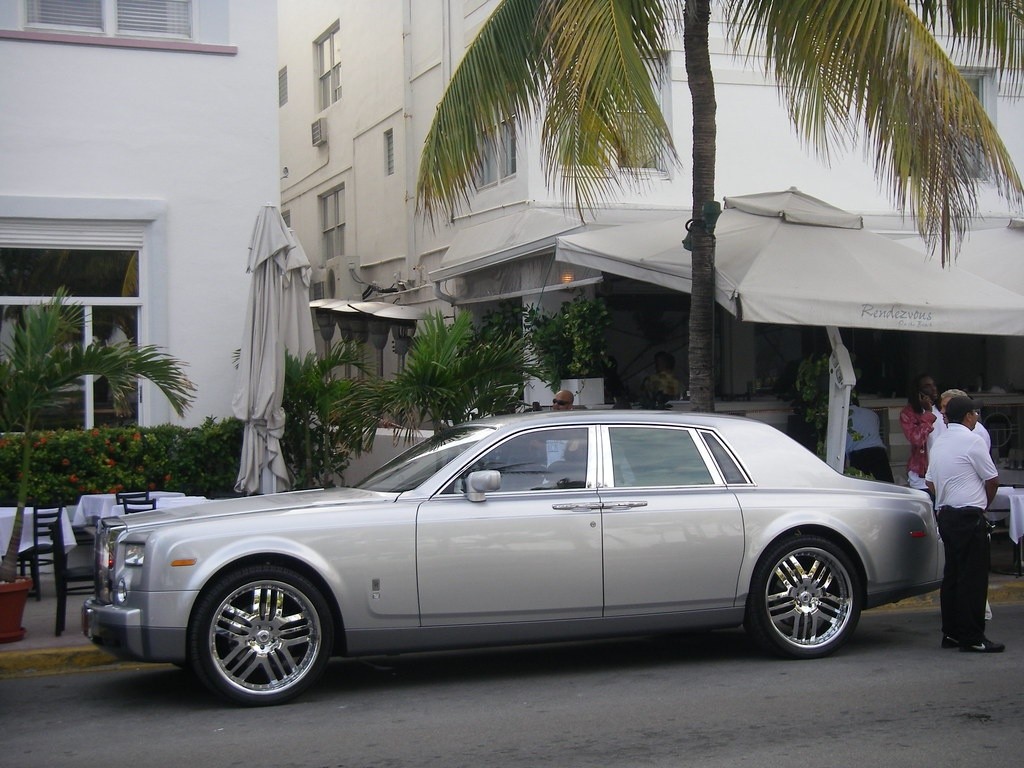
[81,491,186,535]
[986,488,1024,576]
[0,506,67,565]
[110,499,209,514]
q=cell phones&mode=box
[920,392,925,400]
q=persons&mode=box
[848,389,1004,652]
[530,390,585,484]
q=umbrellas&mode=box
[234,202,317,495]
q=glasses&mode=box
[552,398,570,405]
[970,409,980,416]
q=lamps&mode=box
[557,270,576,292]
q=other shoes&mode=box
[984,599,993,620]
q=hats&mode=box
[945,396,985,418]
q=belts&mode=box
[940,505,983,511]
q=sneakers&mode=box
[940,632,966,648]
[958,638,1006,653]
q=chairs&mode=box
[542,461,585,488]
[3,500,64,600]
[115,489,149,504]
[122,495,157,514]
[48,518,95,638]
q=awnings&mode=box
[555,186,1024,337]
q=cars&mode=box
[83,407,948,711]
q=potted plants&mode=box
[0,285,199,646]
[516,294,617,405]
